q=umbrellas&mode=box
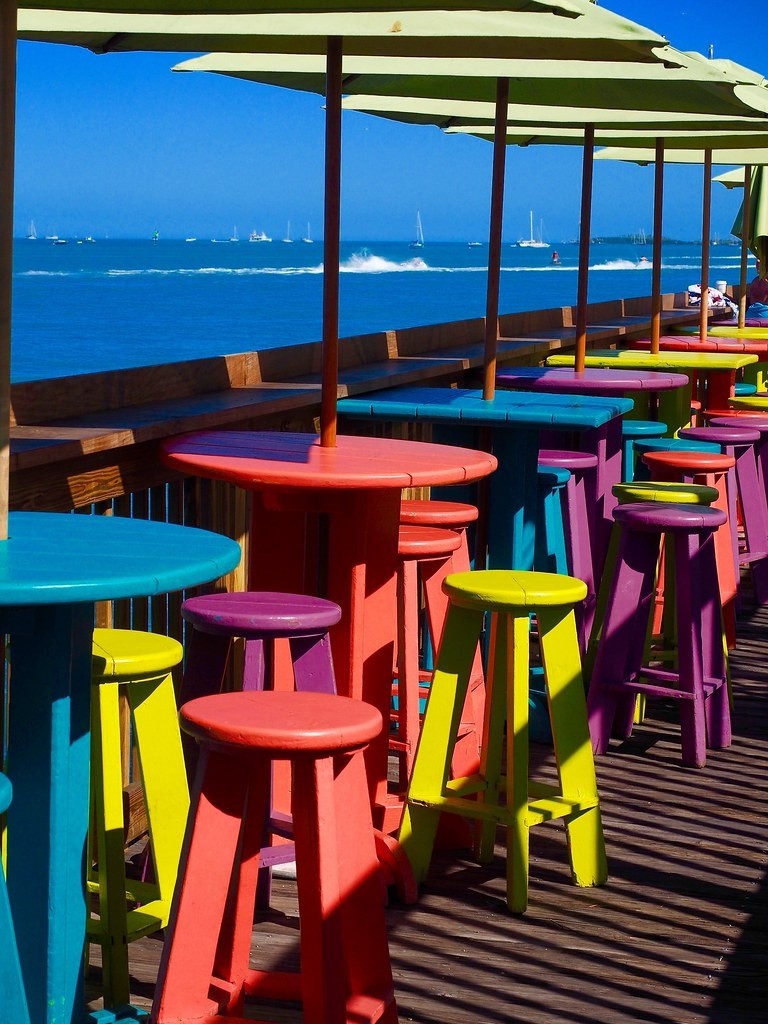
[16,0,767,450]
[730,165,768,261]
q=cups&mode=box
[716,281,727,294]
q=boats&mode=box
[230,225,239,242]
[248,231,272,242]
[186,238,197,242]
[211,238,230,242]
[28,220,97,245]
[467,242,483,247]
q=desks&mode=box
[491,368,689,562]
[0,511,241,1024]
[158,431,499,903]
[336,385,635,742]
[545,317,768,439]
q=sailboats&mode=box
[303,221,315,244]
[510,210,551,248]
[281,219,294,243]
[407,209,426,248]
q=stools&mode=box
[3,627,191,1010]
[382,384,768,767]
[395,570,608,912]
[399,499,488,746]
[150,692,398,1024]
[0,772,31,1024]
[139,592,389,911]
[398,524,486,849]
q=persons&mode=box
[640,255,648,262]
[723,259,768,316]
[551,251,559,263]
